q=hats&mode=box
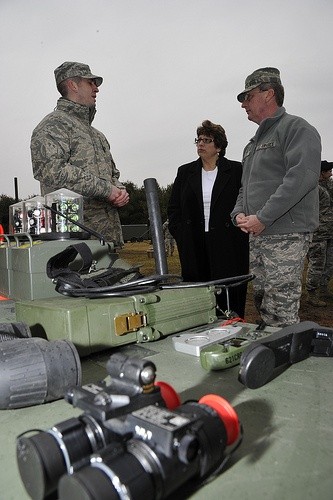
[237,67,280,102]
[54,61,103,87]
[321,160,333,170]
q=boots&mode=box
[319,292,333,301]
[306,290,327,306]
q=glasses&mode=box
[245,89,268,102]
[194,137,214,143]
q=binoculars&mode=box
[17,357,244,500]
[1,321,84,412]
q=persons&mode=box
[30,62,130,252]
[230,68,321,328]
[306,161,333,306]
[163,219,175,257]
[167,120,250,319]
